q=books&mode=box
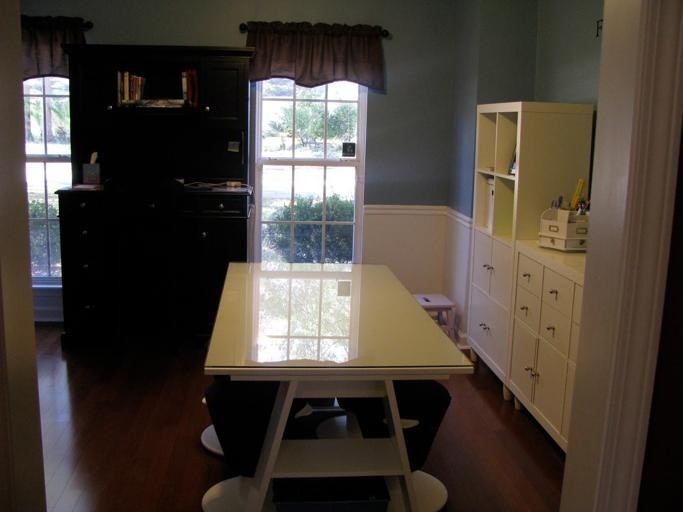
[117,66,198,111]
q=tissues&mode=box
[82,150,101,185]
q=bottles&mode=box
[508,138,517,176]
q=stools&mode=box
[413,293,456,339]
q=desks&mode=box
[200,261,475,512]
